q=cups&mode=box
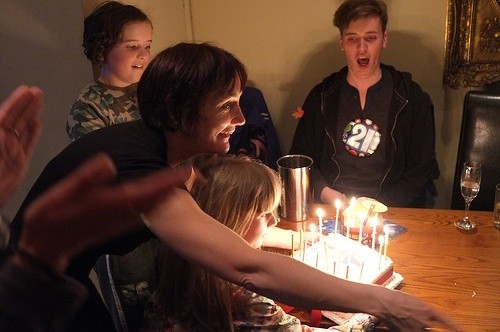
[276,154,313,222]
[492,183,500,229]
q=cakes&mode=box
[339,197,388,239]
[289,231,394,287]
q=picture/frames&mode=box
[443,0,500,87]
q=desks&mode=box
[276,203,500,332]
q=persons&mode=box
[1,87,193,331]
[11,0,461,332]
[287,0,441,208]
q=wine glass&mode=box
[454,159,481,230]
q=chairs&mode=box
[450,88,500,211]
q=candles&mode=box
[346,197,366,242]
[299,227,301,255]
[383,224,388,261]
[316,208,323,239]
[333,260,336,275]
[346,265,349,279]
[323,238,329,268]
[377,235,383,269]
[292,233,294,257]
[302,236,307,260]
[359,261,364,280]
[315,251,319,269]
[372,216,378,250]
[310,224,316,248]
[334,198,340,234]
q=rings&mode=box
[14,128,20,136]
[7,124,14,134]
